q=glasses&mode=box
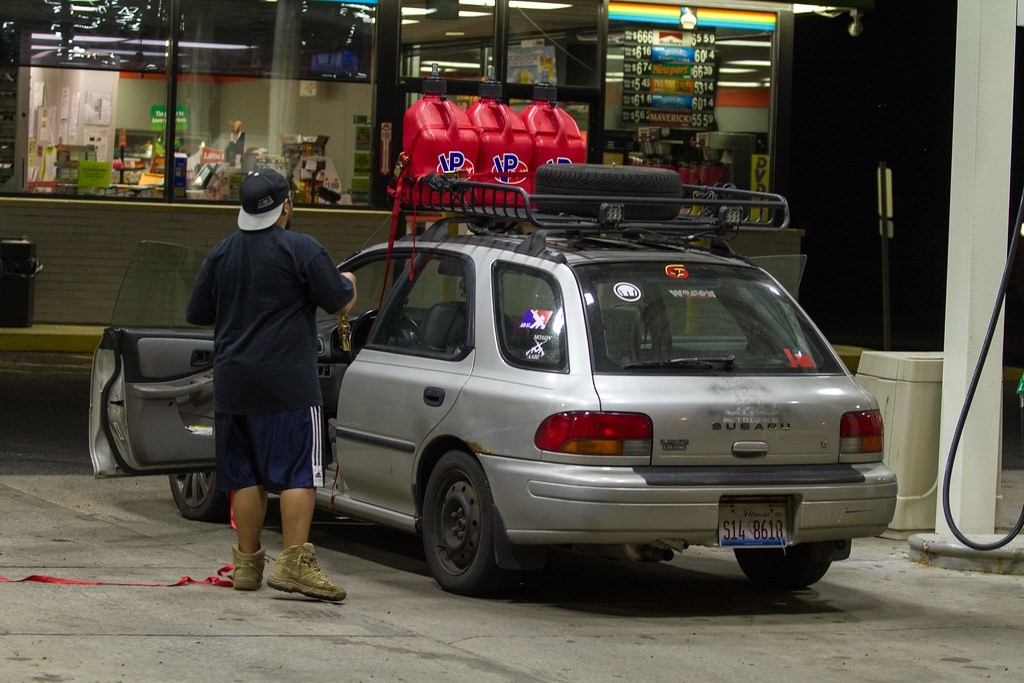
[288,191,296,198]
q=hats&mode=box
[238,169,289,230]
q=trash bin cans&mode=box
[854,350,1005,540]
[0,235,36,328]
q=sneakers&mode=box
[268,542,346,600]
[233,543,265,589]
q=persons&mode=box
[224,120,246,166]
[185,169,358,602]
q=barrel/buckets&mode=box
[403,92,589,204]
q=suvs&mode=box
[89,164,899,592]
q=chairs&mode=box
[418,300,468,351]
